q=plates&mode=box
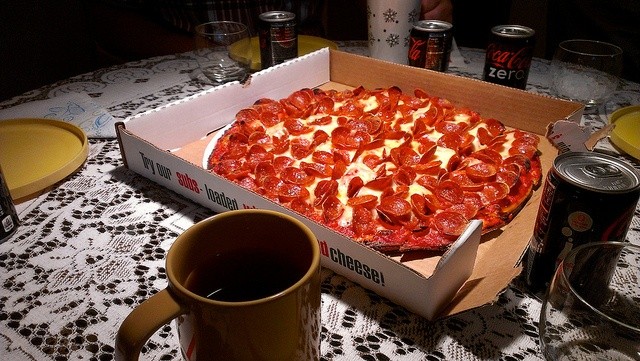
[608,106,640,164]
[228,36,338,72]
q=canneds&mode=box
[408,19,454,71]
[483,22,536,91]
[256,10,298,72]
[524,149,640,304]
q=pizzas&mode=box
[205,86,543,254]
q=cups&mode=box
[540,240,640,361]
[193,21,252,85]
[550,40,622,109]
[114,209,322,361]
